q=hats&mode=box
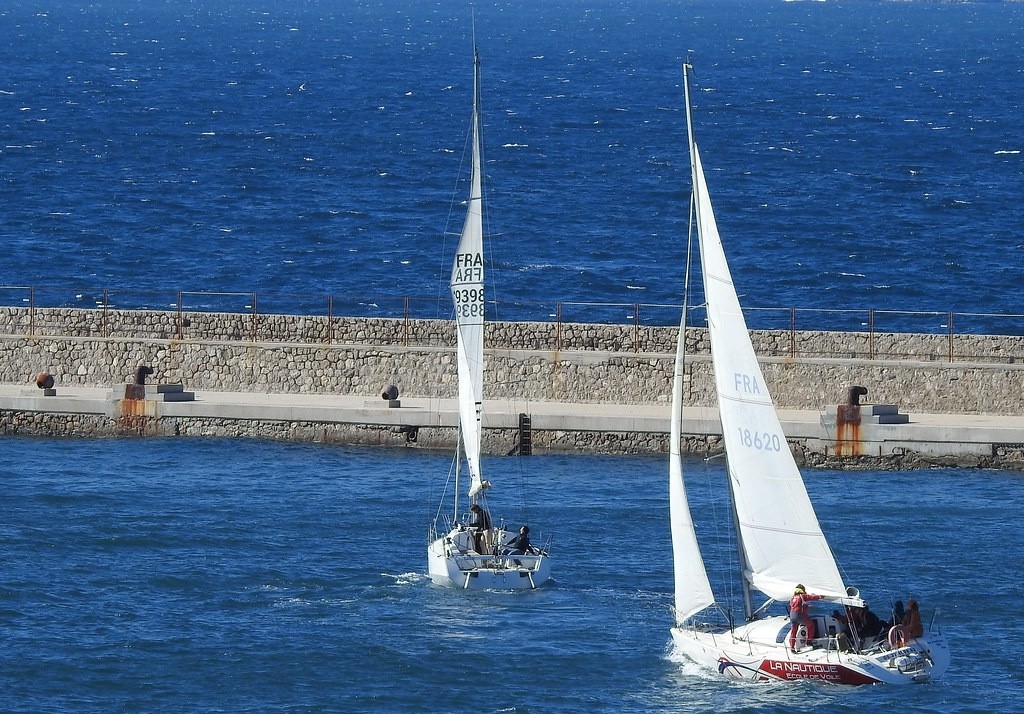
[797,584,805,590]
[908,599,918,608]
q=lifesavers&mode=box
[888,625,911,648]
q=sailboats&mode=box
[671,62,952,687]
[428,7,550,590]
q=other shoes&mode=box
[807,638,815,645]
[790,645,796,653]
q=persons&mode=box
[469,504,494,555]
[500,525,531,556]
[873,599,924,642]
[788,584,826,654]
[836,598,883,640]
